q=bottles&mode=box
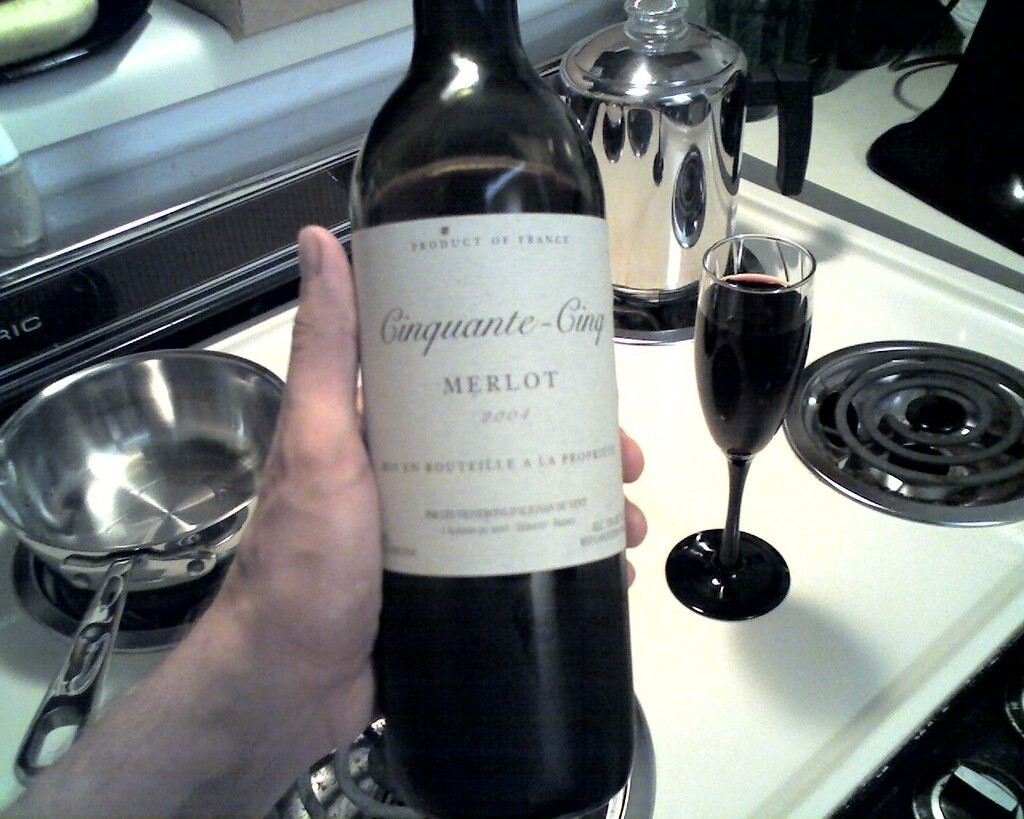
[349,0,635,819]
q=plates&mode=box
[0,0,155,80]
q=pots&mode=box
[1,348,286,787]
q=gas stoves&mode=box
[1,51,1024,818]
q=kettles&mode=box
[559,1,814,319]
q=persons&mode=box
[0,225,382,819]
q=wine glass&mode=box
[664,233,817,622]
